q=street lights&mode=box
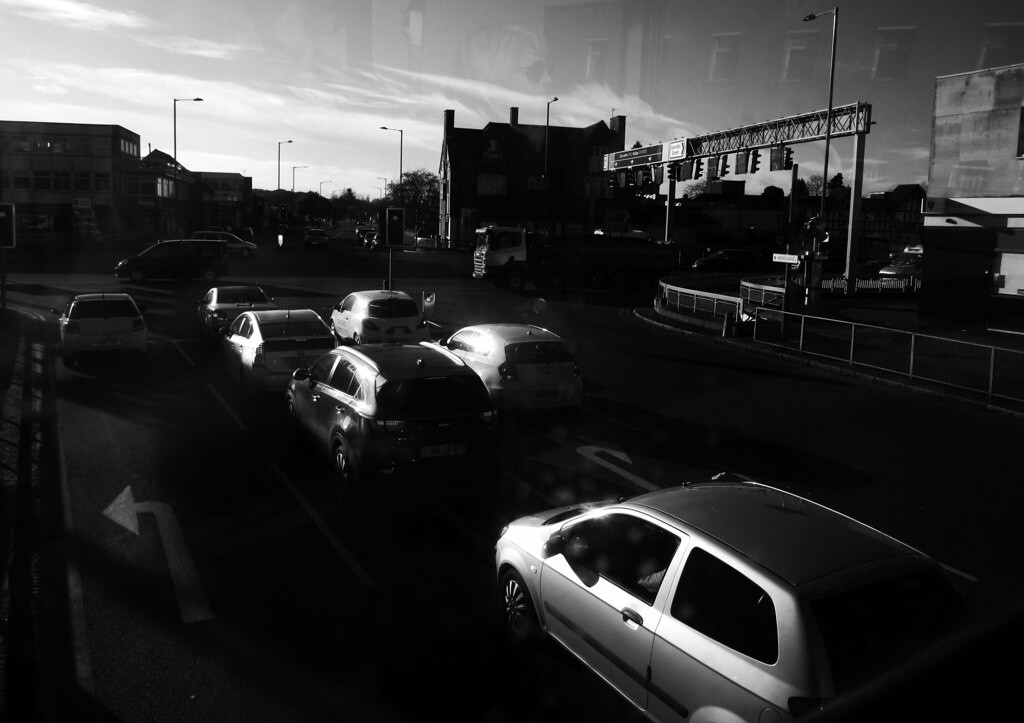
[279,141,292,220]
[173,97,203,217]
[382,127,403,207]
[543,98,558,216]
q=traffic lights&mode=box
[609,159,704,190]
[719,155,730,177]
[750,149,762,173]
[385,208,405,248]
[784,148,794,171]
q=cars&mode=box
[278,342,495,492]
[355,226,389,255]
[330,290,430,345]
[419,322,589,427]
[878,255,922,281]
[494,471,1003,723]
[50,293,147,366]
[218,308,341,398]
[112,240,232,285]
[195,285,278,336]
[190,230,258,257]
